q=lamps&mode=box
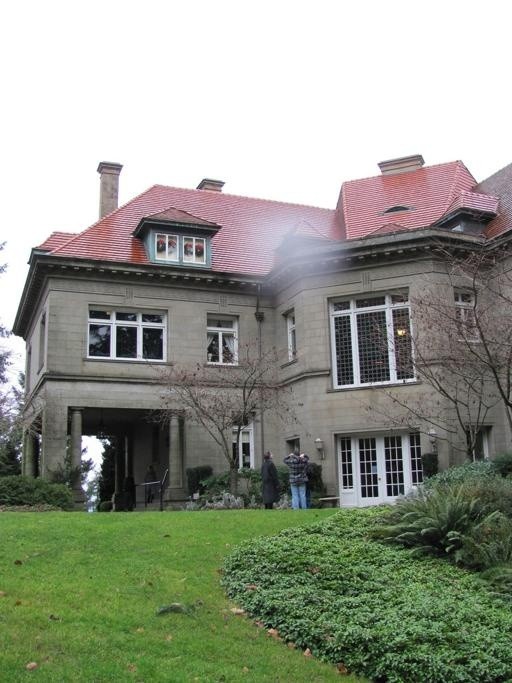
[426,426,438,453]
[313,437,327,460]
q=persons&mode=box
[144,465,157,501]
[282,448,311,510]
[122,472,136,511]
[259,449,280,508]
